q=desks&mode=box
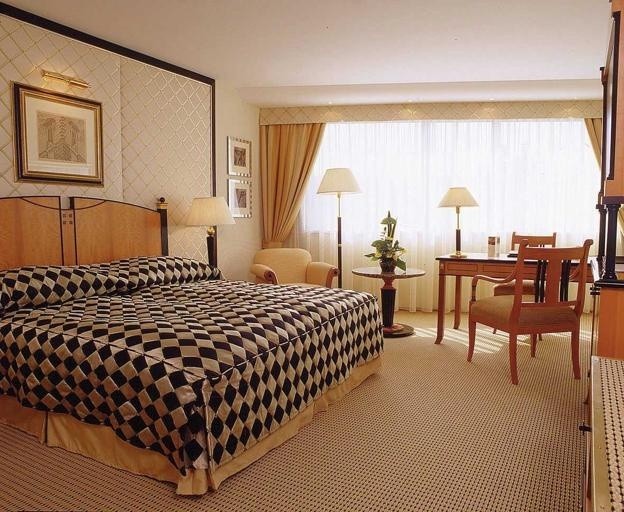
[351,267,426,338]
[434,252,595,345]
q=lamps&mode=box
[185,196,235,269]
[438,187,479,258]
[316,167,360,289]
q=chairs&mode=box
[491,231,556,341]
[250,247,340,289]
[465,239,593,385]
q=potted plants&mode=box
[364,210,407,272]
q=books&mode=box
[507,250,518,257]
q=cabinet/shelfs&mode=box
[584,255,624,421]
[580,356,623,511]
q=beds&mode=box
[0,194,385,497]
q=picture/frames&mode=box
[10,80,105,188]
[226,135,252,178]
[226,179,253,219]
